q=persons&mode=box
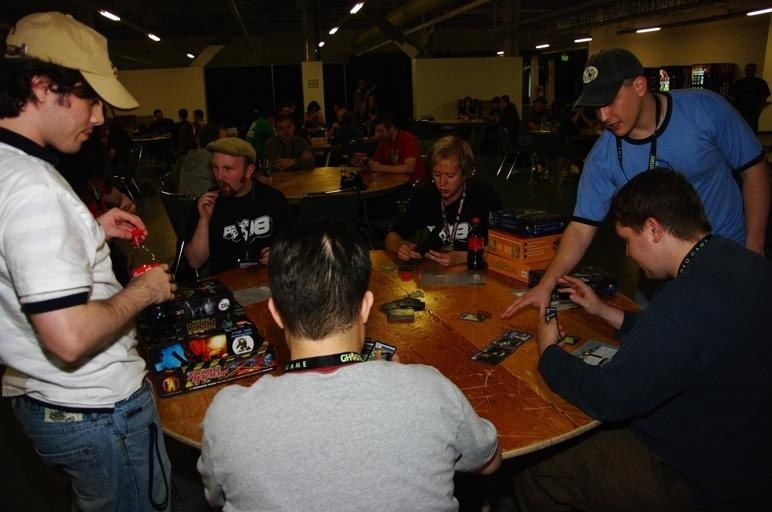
[60,59,768,234]
[181,139,295,272]
[193,218,503,511]
[537,168,770,511]
[1,10,177,511]
[385,136,502,267]
[498,46,772,320]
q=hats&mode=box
[572,47,644,110]
[4,11,141,111]
[206,137,257,163]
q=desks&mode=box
[418,118,496,156]
[136,249,642,459]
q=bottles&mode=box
[128,229,166,326]
[467,216,486,271]
